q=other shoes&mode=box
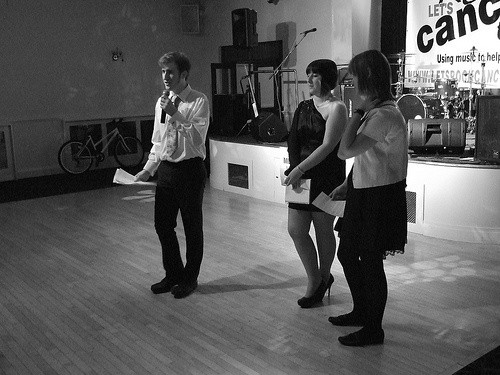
[328,311,365,326]
[170,278,198,299]
[338,326,385,347]
[150,272,187,294]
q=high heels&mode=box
[297,272,335,308]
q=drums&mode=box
[435,78,458,99]
[397,94,426,131]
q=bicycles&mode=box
[57,116,145,176]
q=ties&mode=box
[161,95,180,158]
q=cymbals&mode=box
[388,53,415,58]
[391,63,410,66]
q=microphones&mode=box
[161,89,170,123]
[299,28,317,34]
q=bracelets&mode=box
[297,165,305,175]
[354,108,365,117]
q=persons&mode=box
[131,51,209,298]
[327,49,409,347]
[280,58,346,308]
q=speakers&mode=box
[475,95,500,164]
[334,84,358,120]
[250,111,287,142]
[276,21,297,67]
[231,8,258,47]
[407,118,466,154]
